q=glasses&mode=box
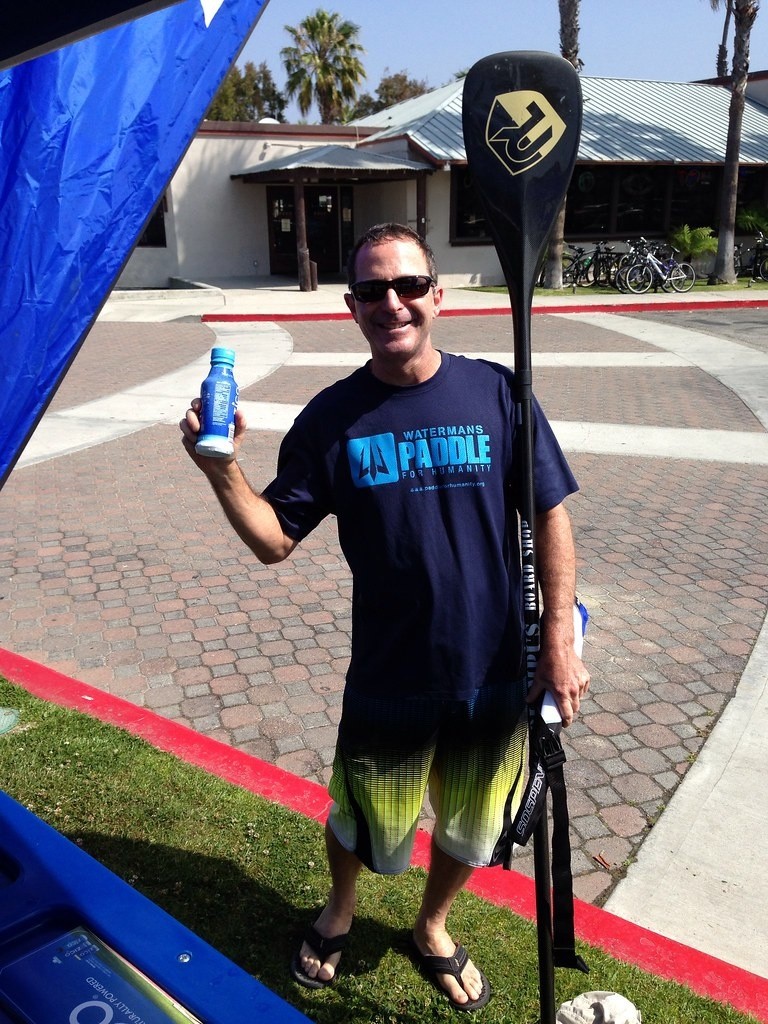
[349,275,435,304]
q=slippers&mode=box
[291,913,355,989]
[406,928,490,1011]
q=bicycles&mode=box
[537,234,699,294]
[733,230,768,287]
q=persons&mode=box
[179,224,591,1011]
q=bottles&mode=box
[195,346,239,459]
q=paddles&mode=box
[461,48,586,1024]
